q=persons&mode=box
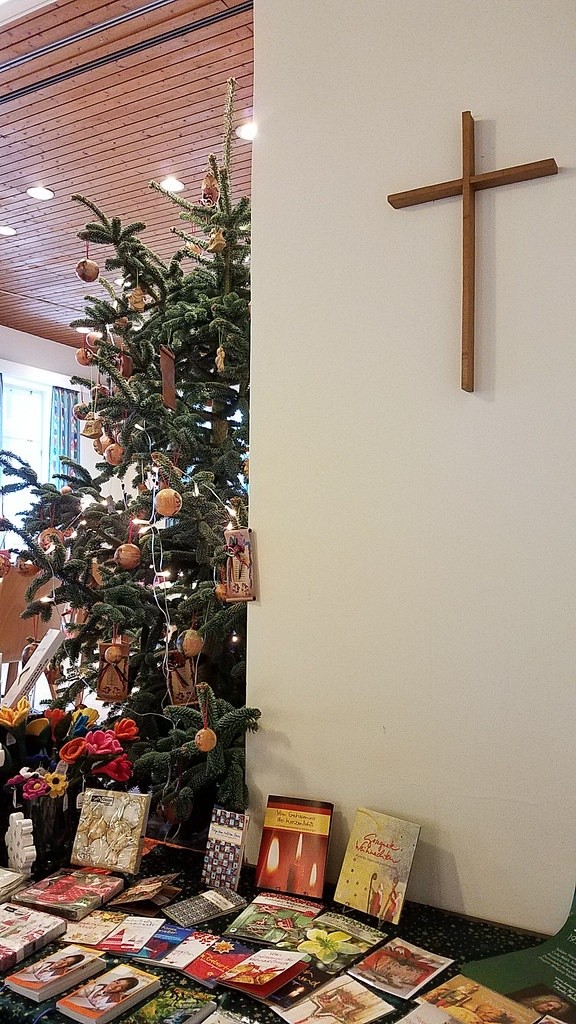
[517,994,570,1018]
[21,954,85,983]
[73,977,139,1011]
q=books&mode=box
[0,789,576,1024]
